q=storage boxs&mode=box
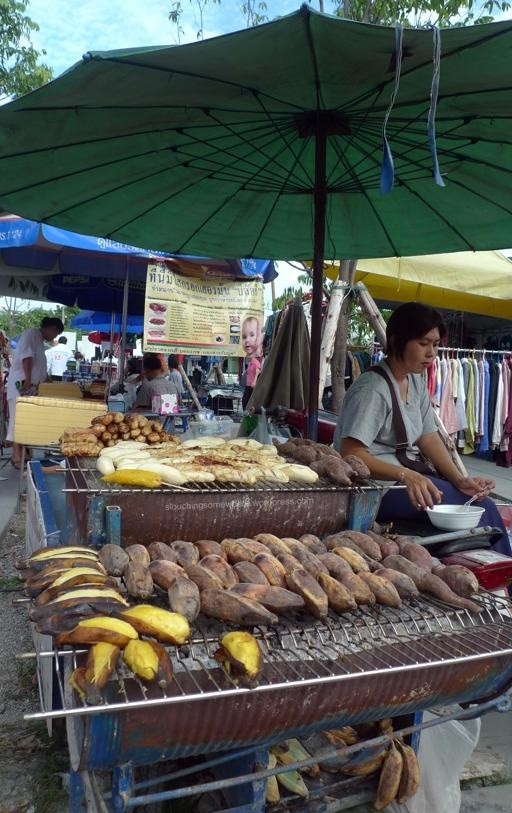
[151,394,179,416]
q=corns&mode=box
[59,409,319,491]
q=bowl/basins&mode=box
[424,502,486,531]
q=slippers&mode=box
[10,458,21,469]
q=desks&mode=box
[211,395,241,417]
[137,411,191,434]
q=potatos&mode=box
[271,427,370,489]
[13,526,490,705]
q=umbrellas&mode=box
[0,1,512,442]
[69,310,144,339]
[0,213,280,393]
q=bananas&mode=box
[261,718,422,813]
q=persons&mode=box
[241,316,259,354]
[239,356,260,423]
[0,316,83,470]
[332,300,511,558]
[109,353,185,436]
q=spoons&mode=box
[460,487,489,513]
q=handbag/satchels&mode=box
[396,443,441,477]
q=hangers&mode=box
[434,346,512,365]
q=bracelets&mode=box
[398,466,409,483]
